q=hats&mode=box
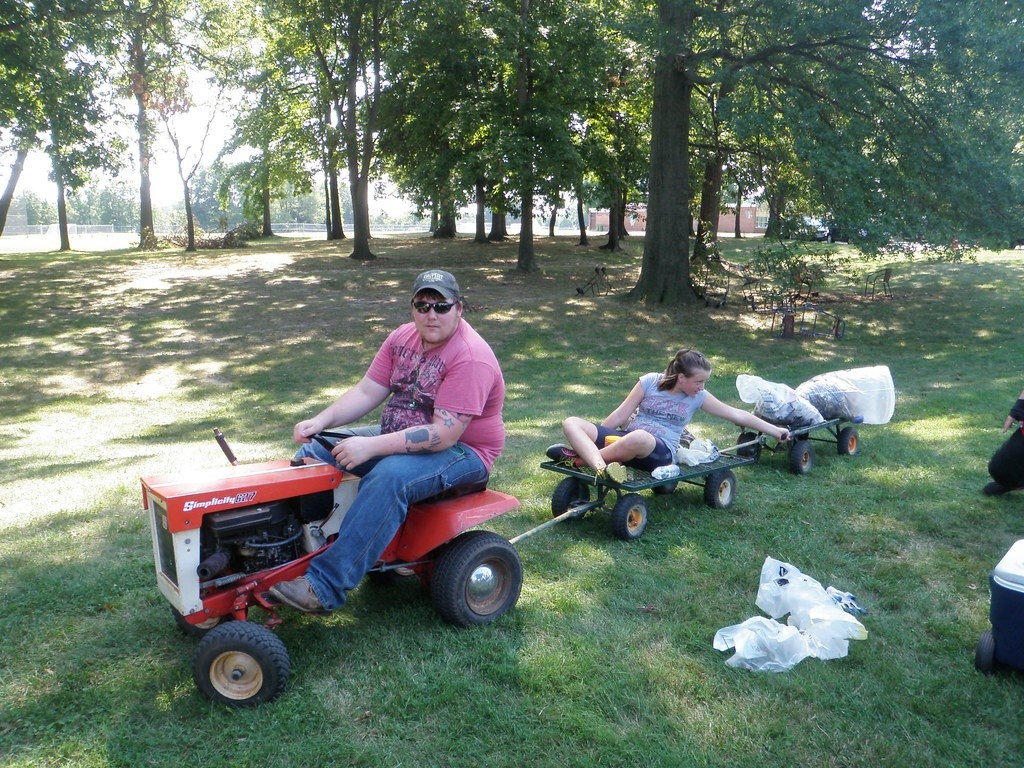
[412,269,460,300]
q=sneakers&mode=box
[545,443,591,469]
[597,461,635,483]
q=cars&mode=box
[781,215,889,245]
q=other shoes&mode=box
[982,479,1024,496]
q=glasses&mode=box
[412,301,458,313]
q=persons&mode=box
[983,386,1024,495]
[268,268,508,615]
[544,346,792,485]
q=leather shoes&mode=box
[269,576,334,618]
